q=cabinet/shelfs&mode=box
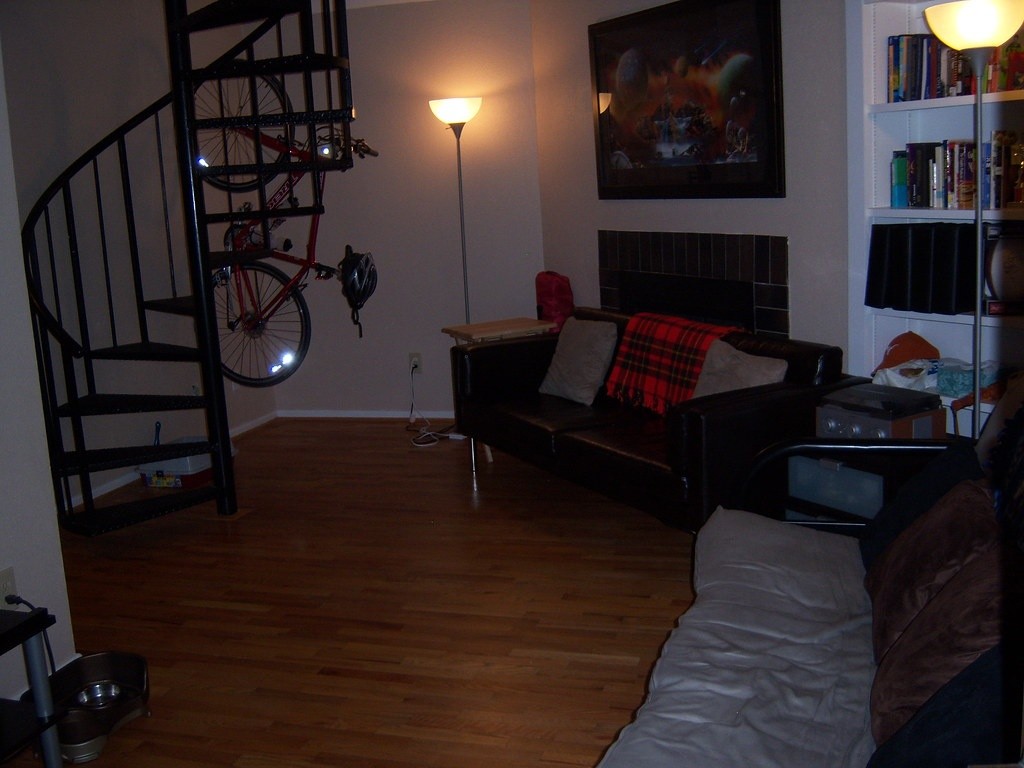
[844,0,1024,442]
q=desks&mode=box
[441,317,558,462]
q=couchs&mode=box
[596,374,1024,768]
[449,308,842,532]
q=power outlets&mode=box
[0,567,20,610]
[408,353,423,373]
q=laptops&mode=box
[442,317,557,343]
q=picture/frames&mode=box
[588,0,786,200]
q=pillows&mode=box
[859,437,1020,768]
[692,340,788,400]
[539,317,618,405]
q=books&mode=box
[888,31,1024,103]
[890,129,1024,210]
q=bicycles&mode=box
[190,54,378,387]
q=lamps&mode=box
[430,98,480,324]
[924,0,1024,441]
